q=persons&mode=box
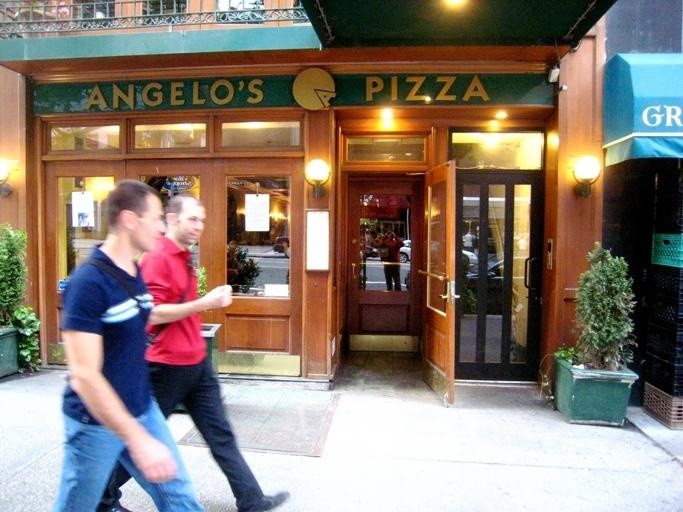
[53,179,212,512]
[461,228,476,253]
[94,193,292,512]
[373,228,405,291]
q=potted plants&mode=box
[0,222,29,379]
[195,267,223,382]
[227,245,264,297]
[554,240,640,428]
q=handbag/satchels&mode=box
[380,247,389,258]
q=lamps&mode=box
[303,159,331,199]
[573,157,602,196]
[0,160,13,196]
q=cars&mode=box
[462,249,478,269]
[364,239,411,264]
[462,249,530,288]
[271,232,290,258]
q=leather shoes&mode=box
[108,504,135,512]
[239,491,288,512]
